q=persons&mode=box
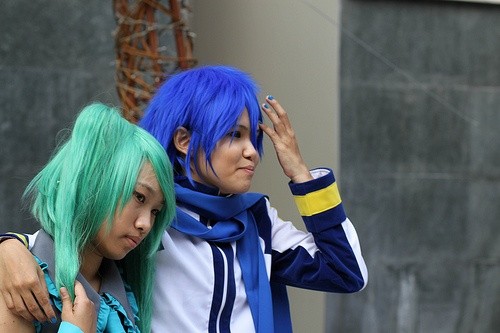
[1,101,175,333]
[1,65,368,333]
